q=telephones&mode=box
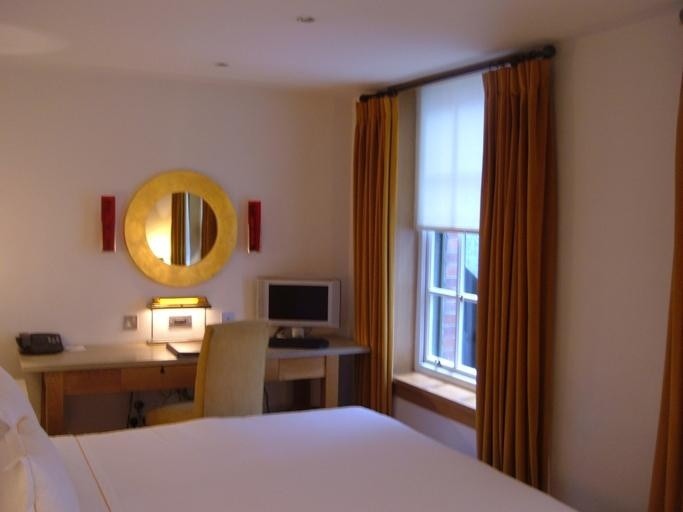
[16,332,64,355]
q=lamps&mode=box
[146,297,212,345]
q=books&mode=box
[166,341,201,358]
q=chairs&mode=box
[145,320,271,426]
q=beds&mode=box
[2,362,577,511]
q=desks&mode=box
[17,335,371,439]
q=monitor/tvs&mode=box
[255,278,342,338]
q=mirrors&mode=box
[123,171,239,288]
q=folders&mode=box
[167,342,200,357]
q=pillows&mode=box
[2,368,73,510]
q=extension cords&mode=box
[127,391,144,428]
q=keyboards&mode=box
[270,336,330,349]
